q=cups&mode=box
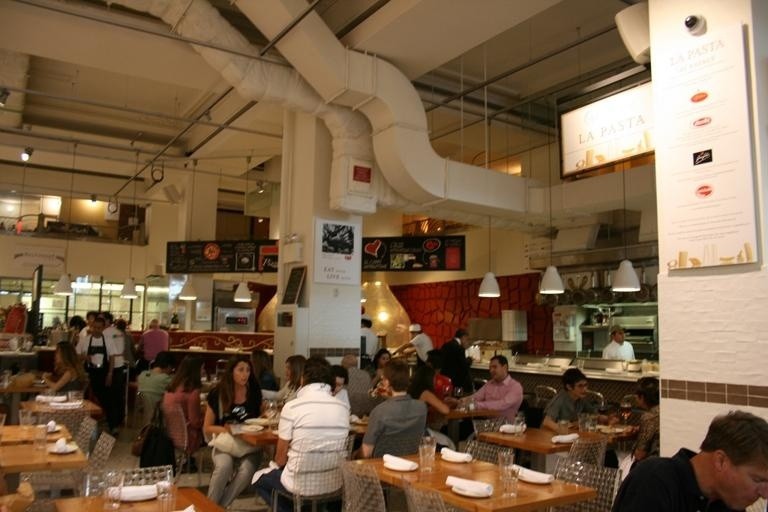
[417,385,634,497]
[1,368,176,512]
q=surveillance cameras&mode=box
[685,14,707,35]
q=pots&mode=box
[535,268,658,301]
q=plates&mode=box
[198,376,219,404]
[240,418,279,432]
[350,415,370,425]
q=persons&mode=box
[41,310,170,441]
[443,356,523,423]
[604,377,660,469]
[539,368,608,435]
[602,326,634,361]
[137,312,472,510]
[610,410,768,512]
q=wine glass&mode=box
[260,398,276,434]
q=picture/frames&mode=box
[281,266,306,305]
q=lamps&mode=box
[610,162,641,293]
[120,179,139,299]
[0,89,10,106]
[178,158,197,301]
[90,194,99,209]
[233,156,252,304]
[538,93,565,294]
[20,146,33,161]
[478,211,501,298]
[52,141,78,297]
